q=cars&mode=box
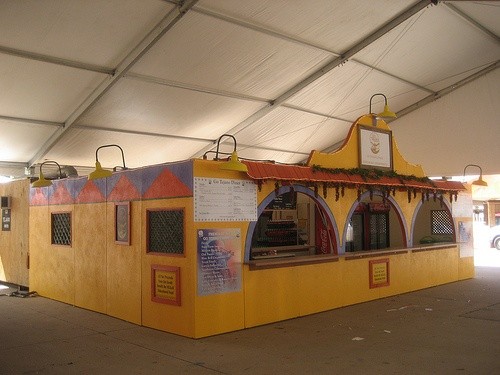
[485,225,500,250]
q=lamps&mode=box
[369,93,397,120]
[214,134,248,174]
[31,160,64,187]
[88,144,127,180]
[462,164,487,187]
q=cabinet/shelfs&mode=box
[261,209,299,248]
